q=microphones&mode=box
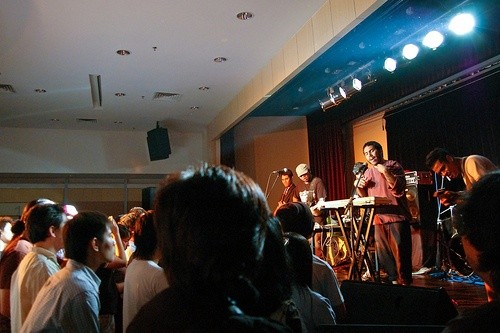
[272,168,288,173]
[356,162,368,175]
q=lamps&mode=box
[330,85,344,105]
[353,69,377,92]
[319,91,335,111]
[339,76,357,100]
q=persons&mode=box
[353,141,412,284]
[0,163,349,333]
[425,147,500,333]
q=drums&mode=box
[447,232,479,278]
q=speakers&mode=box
[404,184,438,225]
[147,128,171,162]
[339,280,459,327]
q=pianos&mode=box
[319,196,389,208]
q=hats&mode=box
[62,205,78,216]
[275,202,312,236]
[295,164,310,178]
